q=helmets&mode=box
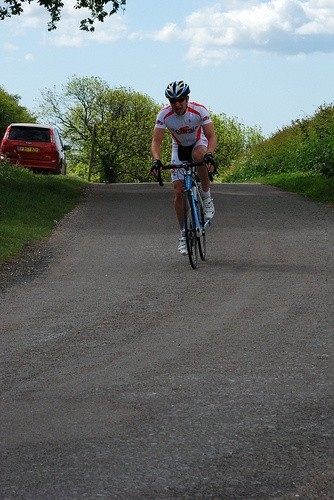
[165,80,190,99]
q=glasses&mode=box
[169,96,187,104]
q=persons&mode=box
[151,81,217,255]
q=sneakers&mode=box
[203,193,216,220]
[178,236,189,256]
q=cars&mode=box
[0,124,70,175]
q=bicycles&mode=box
[150,157,212,269]
